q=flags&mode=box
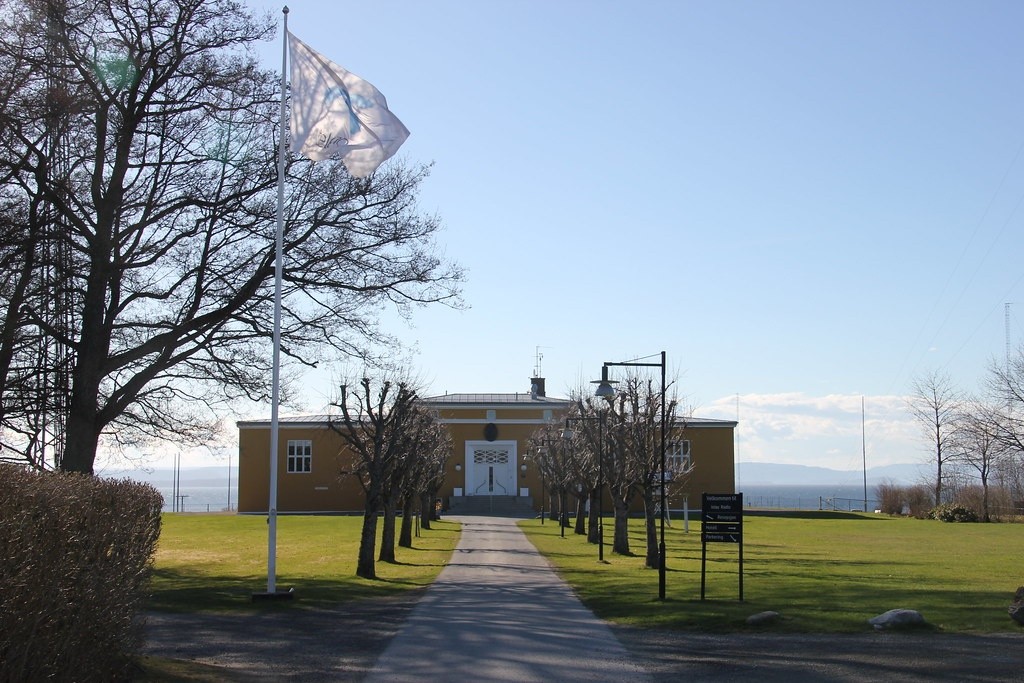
[287,31,410,179]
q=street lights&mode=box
[538,438,566,538]
[591,349,668,599]
[560,410,603,561]
[522,448,545,524]
